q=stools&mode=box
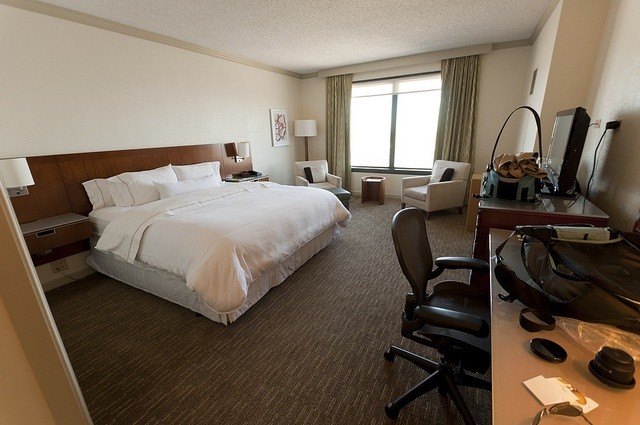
[325,188,351,212]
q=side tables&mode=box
[361,176,386,205]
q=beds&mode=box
[85,181,352,326]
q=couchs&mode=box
[400,159,471,220]
[295,159,342,189]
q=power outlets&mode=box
[593,119,601,129]
[613,119,624,131]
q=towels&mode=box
[493,151,548,179]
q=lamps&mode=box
[294,119,317,161]
[224,141,250,163]
[0,157,36,198]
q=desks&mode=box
[488,227,640,425]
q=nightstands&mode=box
[19,212,97,294]
[223,175,269,182]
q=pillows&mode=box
[171,160,222,193]
[304,164,326,183]
[81,178,116,211]
[107,164,179,208]
[429,161,455,183]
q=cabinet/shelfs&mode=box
[468,172,610,291]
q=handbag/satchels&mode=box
[478,163,544,206]
[491,224,640,333]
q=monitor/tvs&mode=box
[540,104,591,200]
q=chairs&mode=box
[383,206,491,425]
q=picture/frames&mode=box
[269,108,291,147]
